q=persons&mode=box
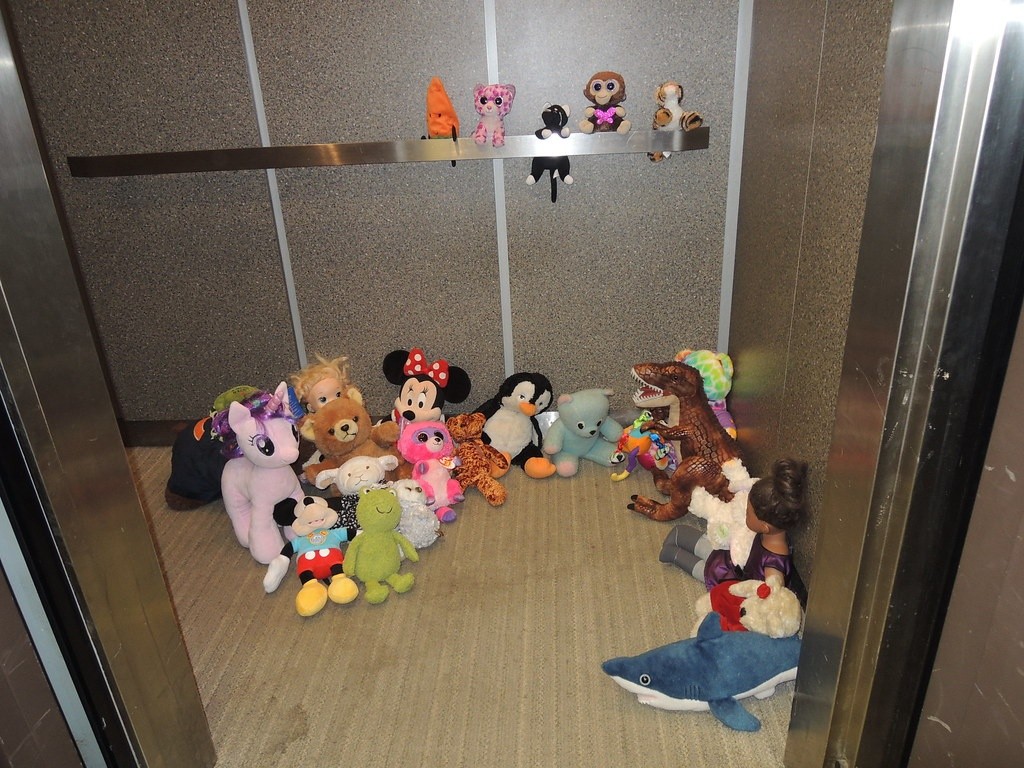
[659,460,808,611]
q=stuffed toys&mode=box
[599,573,801,731]
[165,350,619,618]
[611,347,766,568]
[421,77,460,167]
[468,84,516,148]
[525,101,574,203]
[578,71,631,134]
[648,82,704,163]
